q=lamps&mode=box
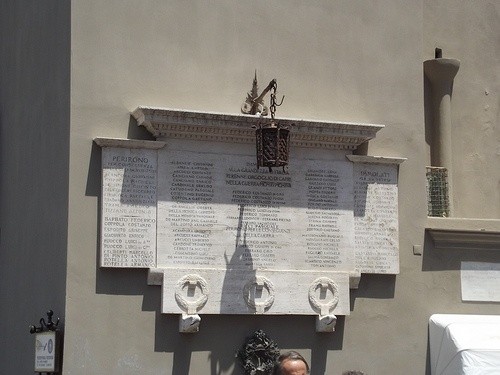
[242,68,292,174]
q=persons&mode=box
[272,351,310,375]
